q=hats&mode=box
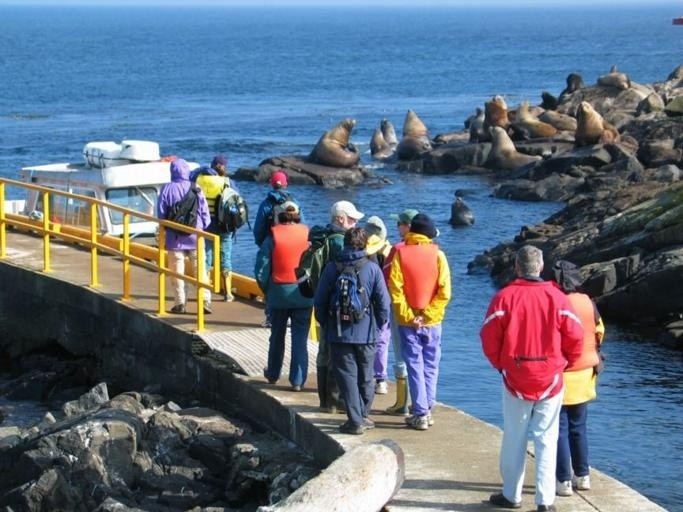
[330,201,365,220]
[410,214,440,240]
[388,209,419,225]
[278,199,299,214]
[271,170,287,188]
[551,260,583,291]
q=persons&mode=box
[190,153,238,304]
[157,158,215,315]
[253,171,314,326]
[548,258,606,497]
[480,246,583,510]
[299,201,451,435]
[256,198,319,390]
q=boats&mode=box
[5,140,199,270]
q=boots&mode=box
[385,375,410,416]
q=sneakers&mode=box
[375,380,389,394]
[339,422,364,435]
[223,294,236,303]
[319,404,333,414]
[263,366,280,384]
[335,408,348,414]
[291,384,304,392]
[556,478,573,495]
[362,415,375,430]
[259,319,272,328]
[536,504,557,512]
[572,473,591,490]
[405,409,433,430]
[490,492,521,508]
[203,300,212,313]
[171,303,185,314]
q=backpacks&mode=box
[293,228,346,299]
[210,176,250,239]
[327,255,375,334]
[165,181,201,237]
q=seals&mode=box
[596,64,631,91]
[448,196,475,230]
[370,74,621,173]
[315,117,361,168]
